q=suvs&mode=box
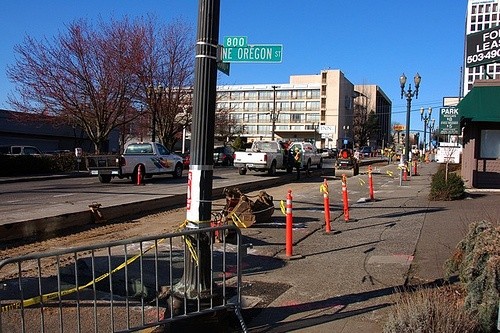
[288,142,323,171]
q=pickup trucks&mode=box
[233,139,304,176]
[2,145,54,157]
[84,141,184,184]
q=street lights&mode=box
[420,105,432,159]
[269,109,280,140]
[426,119,435,154]
[400,72,422,176]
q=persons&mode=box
[353,150,359,162]
[293,148,302,181]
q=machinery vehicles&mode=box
[334,137,359,179]
[354,146,402,166]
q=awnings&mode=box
[456,86,500,122]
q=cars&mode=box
[213,146,234,166]
[321,147,339,159]
